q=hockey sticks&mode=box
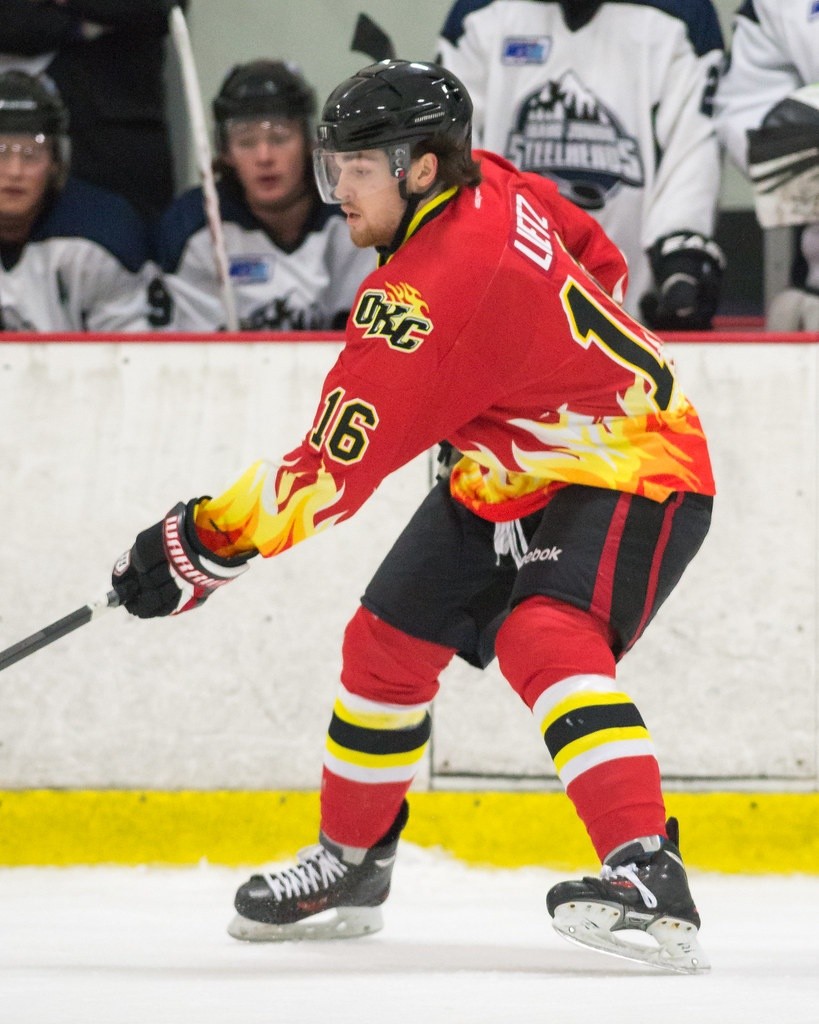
[0,585,130,672]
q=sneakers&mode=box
[545,818,710,974]
[225,797,408,942]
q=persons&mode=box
[0,0,819,332]
[111,59,717,973]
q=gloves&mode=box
[112,496,260,618]
[640,234,726,331]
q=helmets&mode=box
[318,59,473,153]
[0,61,71,135]
[211,60,316,119]
